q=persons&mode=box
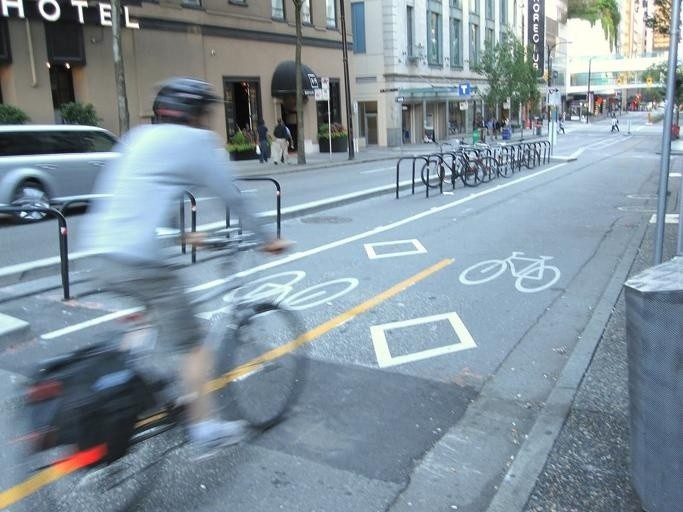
[557,117,565,135]
[76,72,295,456]
[609,116,616,131]
[615,118,620,131]
[255,119,272,163]
[280,119,295,163]
[272,118,289,165]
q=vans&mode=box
[0,123,121,224]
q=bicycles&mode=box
[21,231,313,512]
[421,138,541,187]
[458,251,561,294]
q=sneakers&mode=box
[190,419,249,448]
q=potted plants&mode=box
[225,128,272,160]
[318,122,347,153]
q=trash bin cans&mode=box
[502,128,510,140]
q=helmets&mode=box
[151,76,223,123]
